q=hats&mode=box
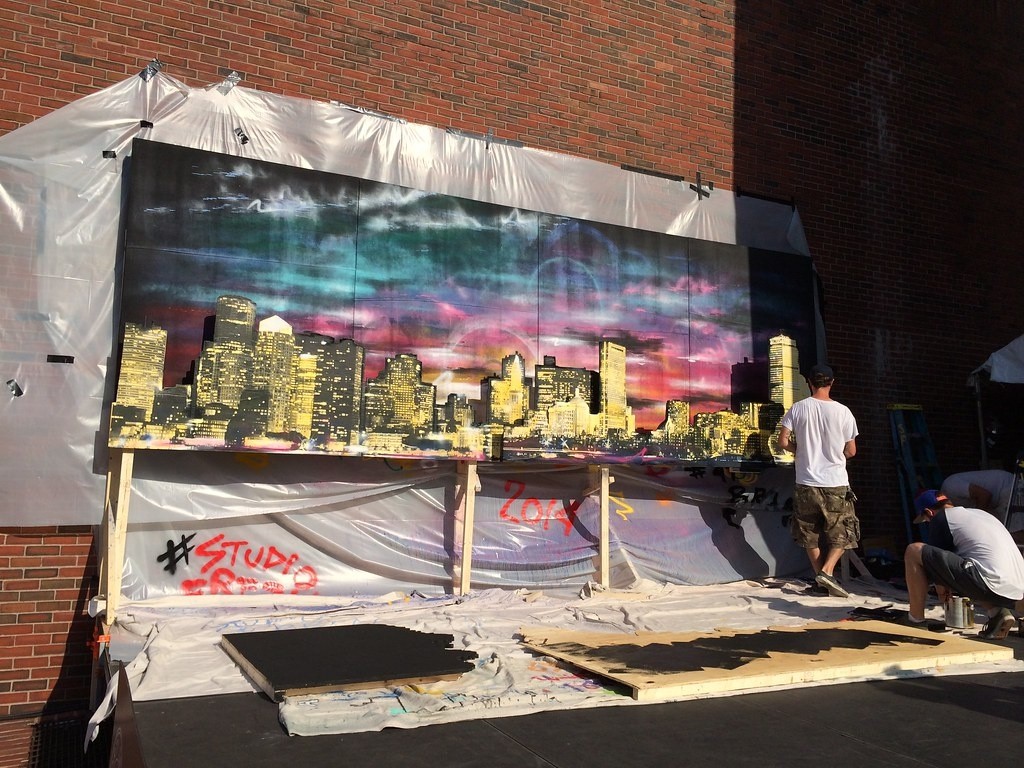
[912,489,948,524]
[808,364,833,380]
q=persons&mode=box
[891,490,1024,640]
[777,363,861,596]
[937,469,1024,534]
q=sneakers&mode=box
[893,612,928,629]
[804,584,829,596]
[978,610,1015,640]
[815,571,850,598]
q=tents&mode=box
[973,336,1024,469]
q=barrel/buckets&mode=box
[943,593,976,630]
